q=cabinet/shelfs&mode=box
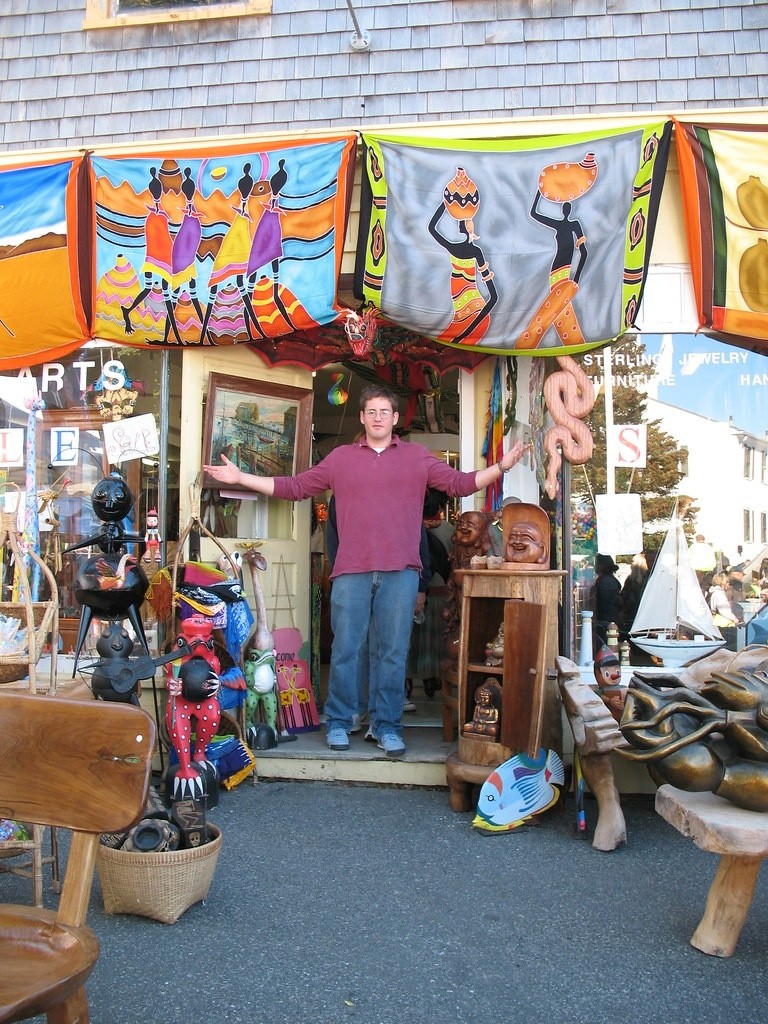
[0,482,61,906]
[451,568,568,765]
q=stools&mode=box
[446,752,497,812]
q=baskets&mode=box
[97,819,222,925]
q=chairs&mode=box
[0,691,156,1024]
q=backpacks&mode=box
[705,589,724,610]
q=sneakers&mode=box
[350,714,363,734]
[364,725,375,741]
[403,698,417,712]
[376,734,406,755]
[327,728,349,750]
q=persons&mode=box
[484,621,504,666]
[204,384,531,756]
[620,554,650,628]
[311,494,458,743]
[464,687,498,736]
[503,522,548,564]
[689,535,768,624]
[594,643,629,725]
[442,511,488,639]
[488,496,522,557]
[586,553,622,647]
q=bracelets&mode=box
[498,461,511,472]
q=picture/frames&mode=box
[201,370,315,491]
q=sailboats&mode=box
[629,500,728,667]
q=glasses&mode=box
[363,411,393,420]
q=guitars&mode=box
[106,644,192,694]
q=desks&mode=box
[653,783,768,957]
